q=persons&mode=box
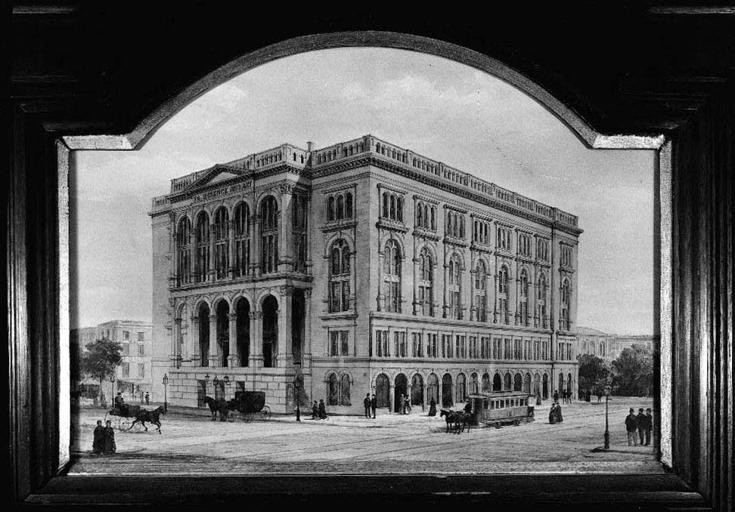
[428,396,438,416]
[371,394,378,419]
[92,419,104,452]
[395,392,411,414]
[364,393,371,418]
[548,388,573,424]
[311,400,318,419]
[462,398,474,414]
[318,398,329,420]
[115,391,123,406]
[144,391,150,405]
[103,420,117,454]
[625,405,654,447]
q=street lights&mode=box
[294,379,301,421]
[602,382,612,449]
[163,373,169,413]
[213,375,220,399]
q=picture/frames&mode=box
[0,0,735,510]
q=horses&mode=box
[439,409,471,434]
[127,406,165,435]
[203,395,229,422]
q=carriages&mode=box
[103,404,168,435]
[204,390,272,423]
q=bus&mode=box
[468,389,536,430]
